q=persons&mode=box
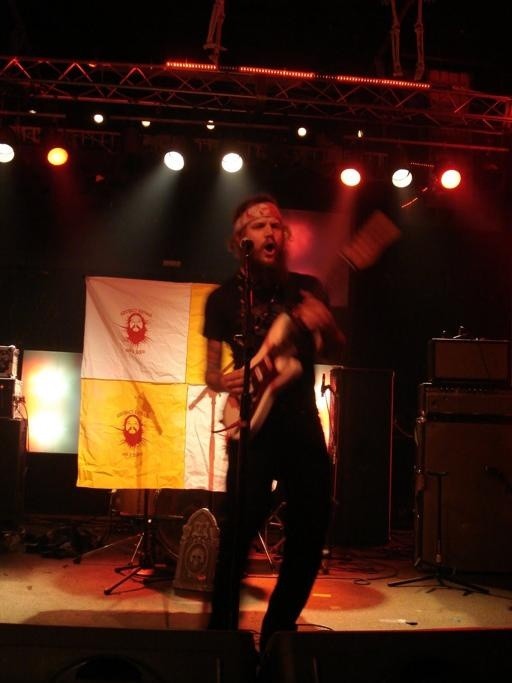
[201,196,348,651]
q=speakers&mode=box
[0,378,25,419]
[0,417,27,532]
[328,366,395,549]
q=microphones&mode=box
[239,238,254,251]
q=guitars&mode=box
[224,211,401,440]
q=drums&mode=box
[110,489,156,518]
[152,489,228,560]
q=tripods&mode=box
[387,472,489,594]
[74,489,178,595]
